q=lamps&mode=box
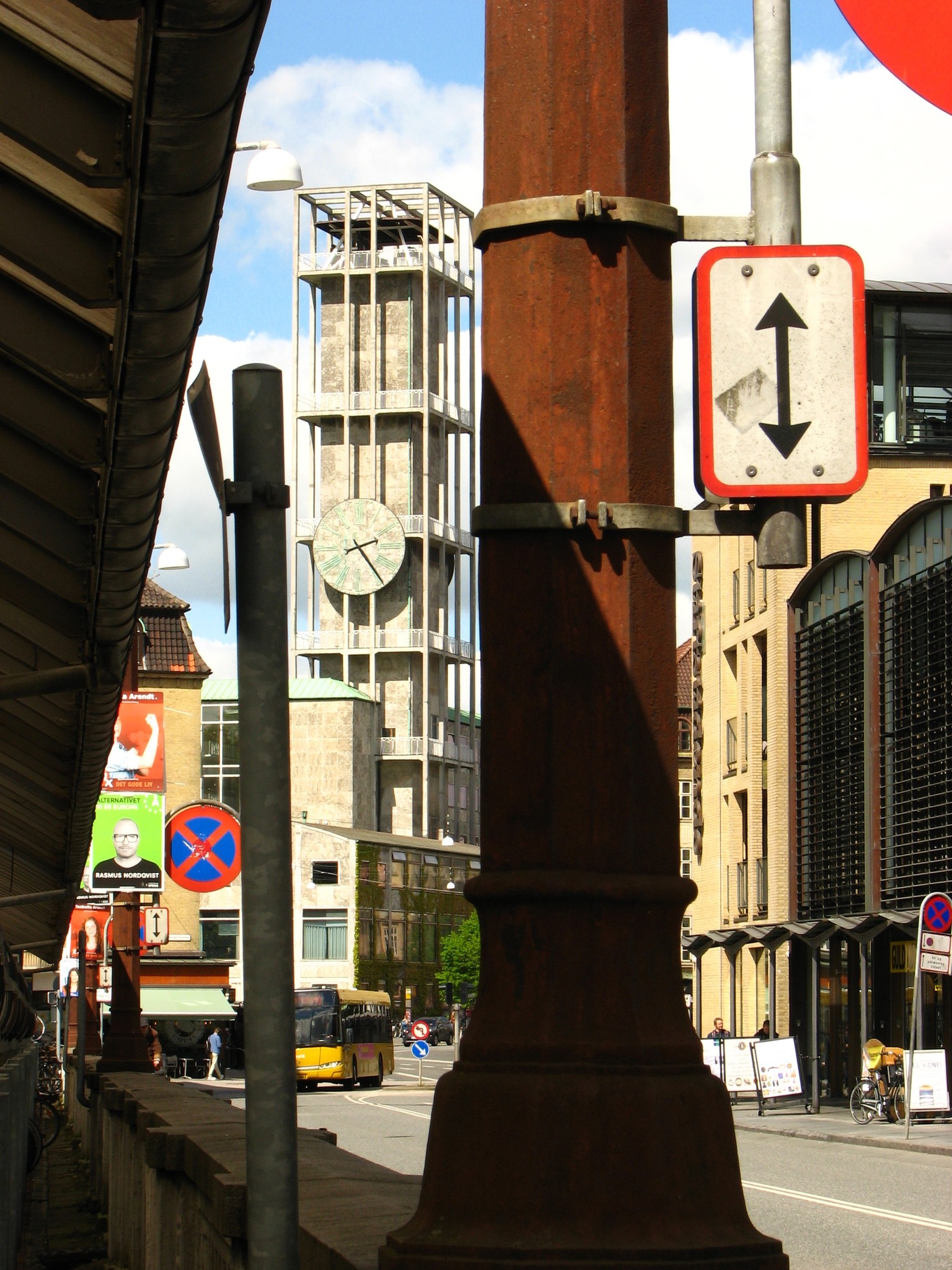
[154,544,189,569]
[447,868,456,889]
[236,140,303,191]
[441,814,453,846]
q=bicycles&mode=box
[25,1012,64,1176]
[391,1019,412,1039]
[849,1051,918,1125]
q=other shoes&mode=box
[220,1074,224,1079]
[207,1076,216,1080]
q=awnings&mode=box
[104,987,238,1022]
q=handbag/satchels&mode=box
[205,1036,211,1053]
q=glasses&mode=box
[114,834,138,842]
[83,874,89,879]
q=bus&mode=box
[294,982,394,1092]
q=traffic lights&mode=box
[47,992,57,1004]
[460,982,475,1005]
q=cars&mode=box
[402,1016,453,1047]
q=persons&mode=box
[708,1018,730,1038]
[754,1020,779,1040]
[71,917,104,959]
[92,818,162,888]
[140,1017,162,1075]
[60,968,79,996]
[75,866,104,905]
[206,1027,224,1081]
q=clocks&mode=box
[312,499,406,596]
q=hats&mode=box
[140,1016,149,1027]
